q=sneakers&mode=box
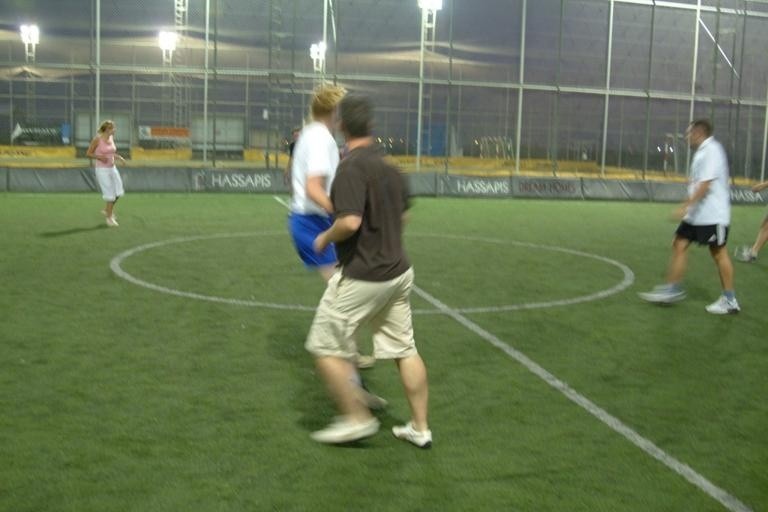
[392,421,435,448]
[106,216,118,226]
[705,294,741,316]
[312,354,388,443]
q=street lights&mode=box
[416,0,443,169]
[17,21,43,121]
[155,28,179,127]
[308,40,328,93]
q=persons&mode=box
[287,128,299,164]
[736,174,766,266]
[86,120,127,228]
[636,120,740,314]
[301,91,437,448]
[289,78,390,412]
